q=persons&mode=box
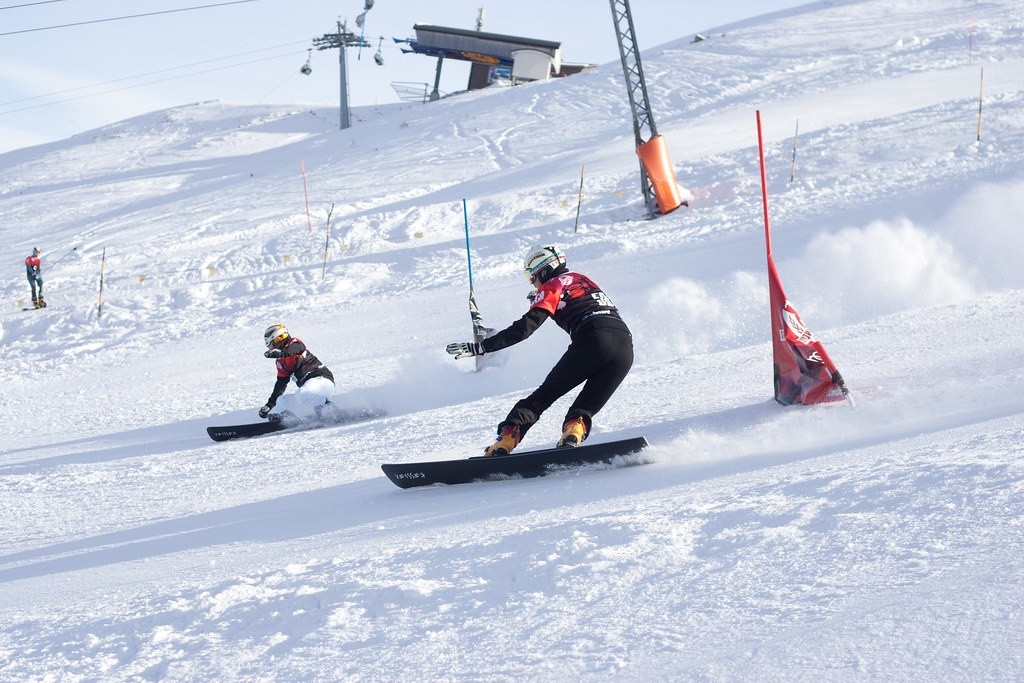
[447,244,634,457]
[259,324,335,428]
[25,246,46,309]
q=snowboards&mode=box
[380,436,652,489]
[22,305,52,311]
[206,408,387,442]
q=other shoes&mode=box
[35,303,40,309]
[39,301,46,308]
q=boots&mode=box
[482,425,521,456]
[555,417,588,448]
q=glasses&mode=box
[524,253,565,284]
[265,328,288,347]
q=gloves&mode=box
[446,342,484,360]
[264,348,282,358]
[259,403,274,418]
[33,274,39,279]
[526,290,538,302]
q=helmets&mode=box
[264,324,290,350]
[34,247,41,255]
[524,243,566,275]
